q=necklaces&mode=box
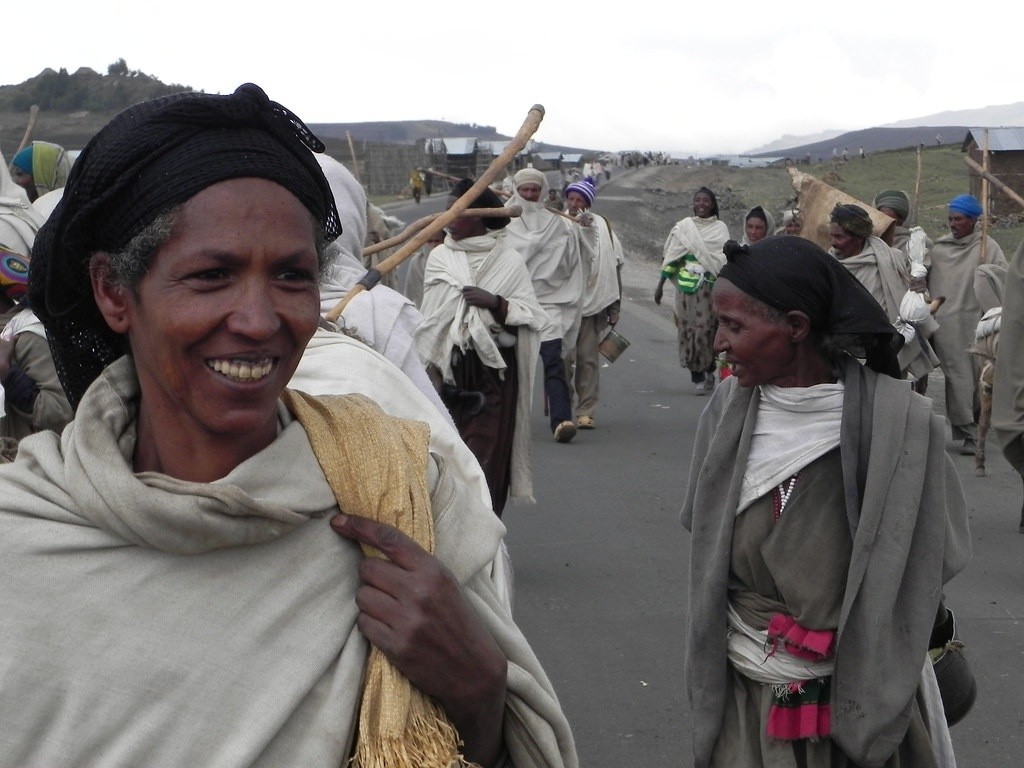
[774,471,799,522]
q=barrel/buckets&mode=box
[930,606,977,727]
[598,329,631,363]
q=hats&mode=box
[451,178,509,231]
[949,194,983,218]
[832,204,873,238]
[565,176,595,207]
[875,190,909,219]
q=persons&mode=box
[871,189,935,273]
[794,152,812,165]
[312,149,460,440]
[926,194,1008,456]
[503,168,599,443]
[990,235,1024,534]
[582,156,611,184]
[827,201,940,394]
[544,180,625,429]
[777,209,803,236]
[860,146,864,157]
[843,148,848,161]
[655,186,730,396]
[615,151,671,169]
[739,206,776,247]
[420,179,551,520]
[936,132,941,146]
[0,83,579,768]
[919,138,924,148]
[0,140,81,464]
[678,235,973,768]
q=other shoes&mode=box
[578,415,593,428]
[961,438,977,454]
[554,420,576,442]
